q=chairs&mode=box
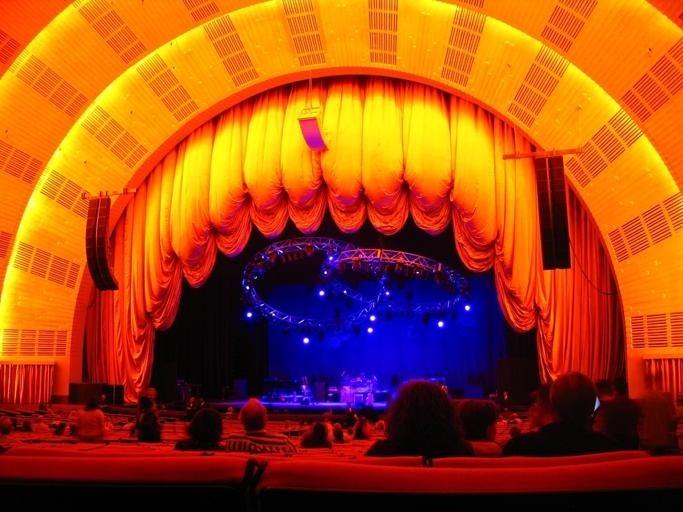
[0,397,683,512]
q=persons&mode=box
[172,408,224,451]
[224,397,298,456]
[0,405,76,452]
[35,401,51,417]
[75,400,106,442]
[298,423,332,449]
[279,417,315,431]
[185,396,207,410]
[128,396,163,443]
[49,395,63,414]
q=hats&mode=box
[187,409,222,442]
